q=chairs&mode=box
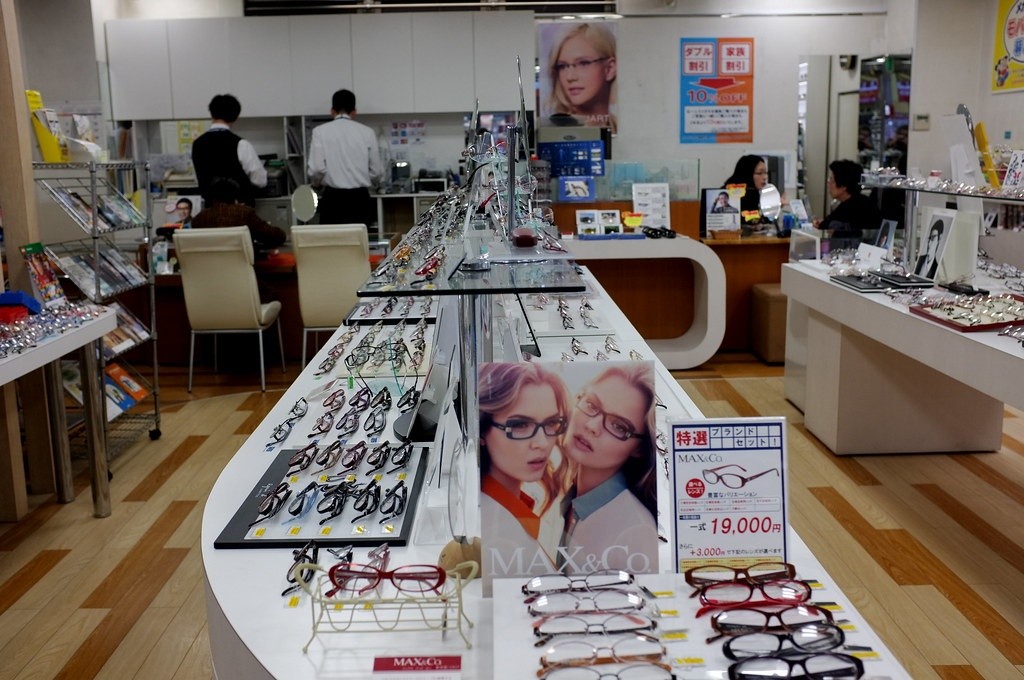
[291,222,372,372]
[172,223,289,394]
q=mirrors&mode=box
[759,183,791,238]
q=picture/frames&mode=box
[912,207,958,282]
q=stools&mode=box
[754,282,788,363]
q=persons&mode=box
[552,363,661,576]
[478,361,571,578]
[811,159,884,258]
[543,19,617,136]
[305,88,383,241]
[190,93,268,210]
[856,115,909,222]
[172,198,194,227]
[191,175,287,306]
[720,153,788,231]
[709,191,739,213]
[914,218,944,281]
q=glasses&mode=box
[818,166,1024,346]
[489,413,569,440]
[557,56,609,71]
[248,140,865,679]
[574,392,645,442]
[0,298,108,359]
[928,235,937,242]
[753,171,768,175]
[702,464,780,489]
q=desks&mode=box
[558,231,727,371]
[54,232,402,371]
[781,255,1024,457]
[199,265,917,680]
[700,237,821,358]
[0,301,118,523]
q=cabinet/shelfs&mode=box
[104,9,541,122]
[148,177,448,246]
[31,160,164,442]
